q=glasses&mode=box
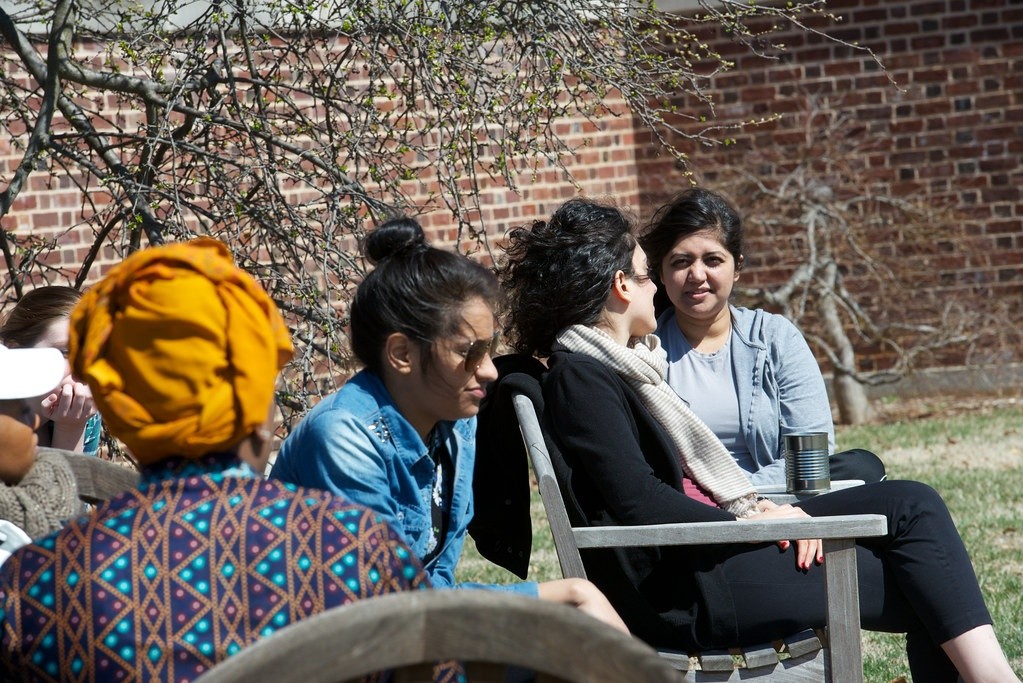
[405,332,501,372]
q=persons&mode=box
[266,216,634,639]
[1,236,438,681]
[631,186,890,515]
[496,198,1023,682]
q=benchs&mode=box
[514,391,887,683]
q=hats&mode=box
[0,344,65,400]
[66,235,297,458]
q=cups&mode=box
[784,430,831,495]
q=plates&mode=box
[0,347,65,400]
